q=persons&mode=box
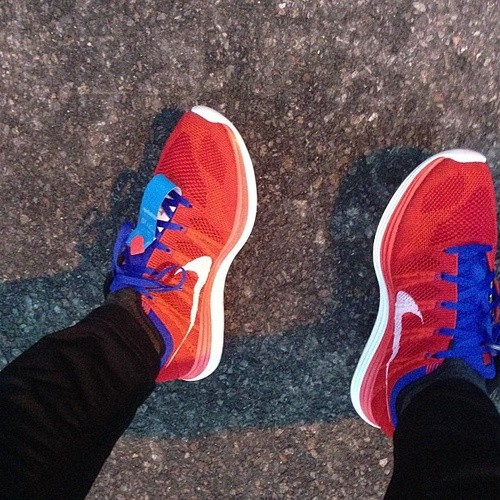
[0,105,500,500]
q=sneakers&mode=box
[108,105,257,384]
[350,148,500,438]
